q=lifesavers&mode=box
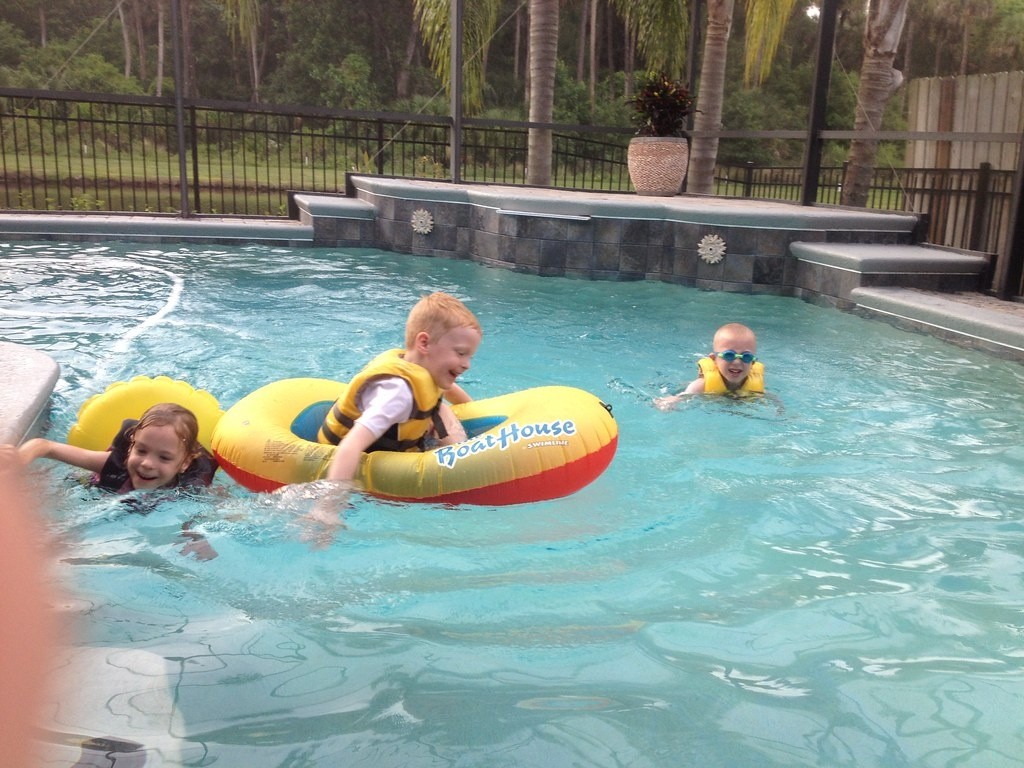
[68,373,229,482]
[211,377,619,503]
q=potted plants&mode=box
[622,69,704,197]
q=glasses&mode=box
[714,350,758,363]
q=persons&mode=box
[315,291,482,480]
[685,322,764,393]
[17,402,219,485]
